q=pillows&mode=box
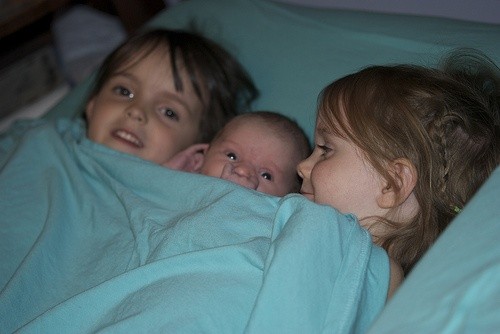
[148,0,460,149]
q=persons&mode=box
[296,44,500,305]
[82,22,259,165]
[161,109,313,197]
